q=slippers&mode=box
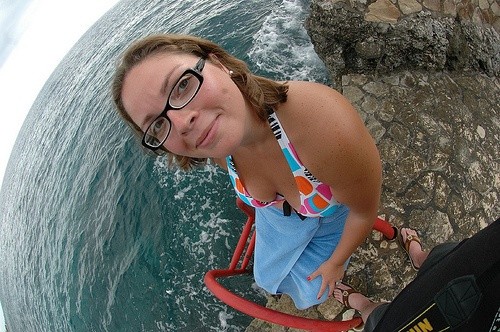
[398,227,424,271]
[332,282,374,313]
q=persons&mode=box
[111,33,384,312]
[332,217,500,332]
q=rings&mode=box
[335,279,342,286]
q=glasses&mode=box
[141,56,208,151]
